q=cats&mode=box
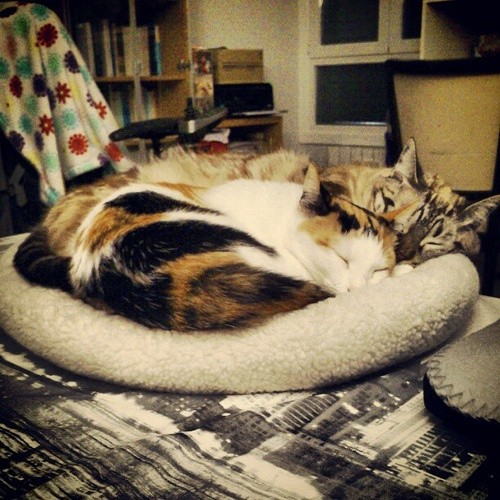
[13,136,500,290]
[68,161,424,334]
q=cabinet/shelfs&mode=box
[24,1,194,155]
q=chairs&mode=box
[385,56,499,205]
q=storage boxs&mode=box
[209,47,263,84]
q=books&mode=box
[76,17,163,79]
[102,85,161,128]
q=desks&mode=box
[1,232,499,498]
